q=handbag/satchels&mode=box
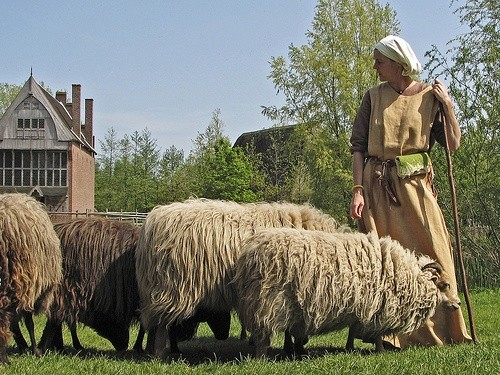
[395,152,431,178]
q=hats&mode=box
[375,35,421,77]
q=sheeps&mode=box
[0,192,461,366]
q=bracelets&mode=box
[352,185,364,191]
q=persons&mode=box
[351,34,473,348]
